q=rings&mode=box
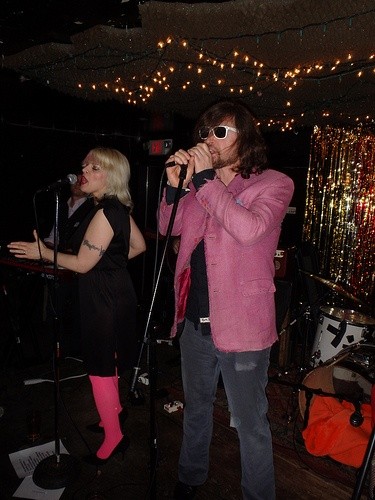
[24,251,25,254]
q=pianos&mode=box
[0,248,76,369]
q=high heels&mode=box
[87,408,129,432]
[87,434,131,467]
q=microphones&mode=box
[39,174,78,193]
[350,411,364,427]
[165,149,210,168]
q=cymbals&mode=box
[299,269,364,305]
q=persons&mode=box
[157,101,295,500]
[45,171,93,252]
[7,147,146,469]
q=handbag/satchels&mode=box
[300,388,374,469]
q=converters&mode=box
[130,388,146,406]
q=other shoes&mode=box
[171,482,197,500]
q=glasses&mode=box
[198,125,240,140]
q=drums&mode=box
[306,302,375,368]
[297,343,375,425]
